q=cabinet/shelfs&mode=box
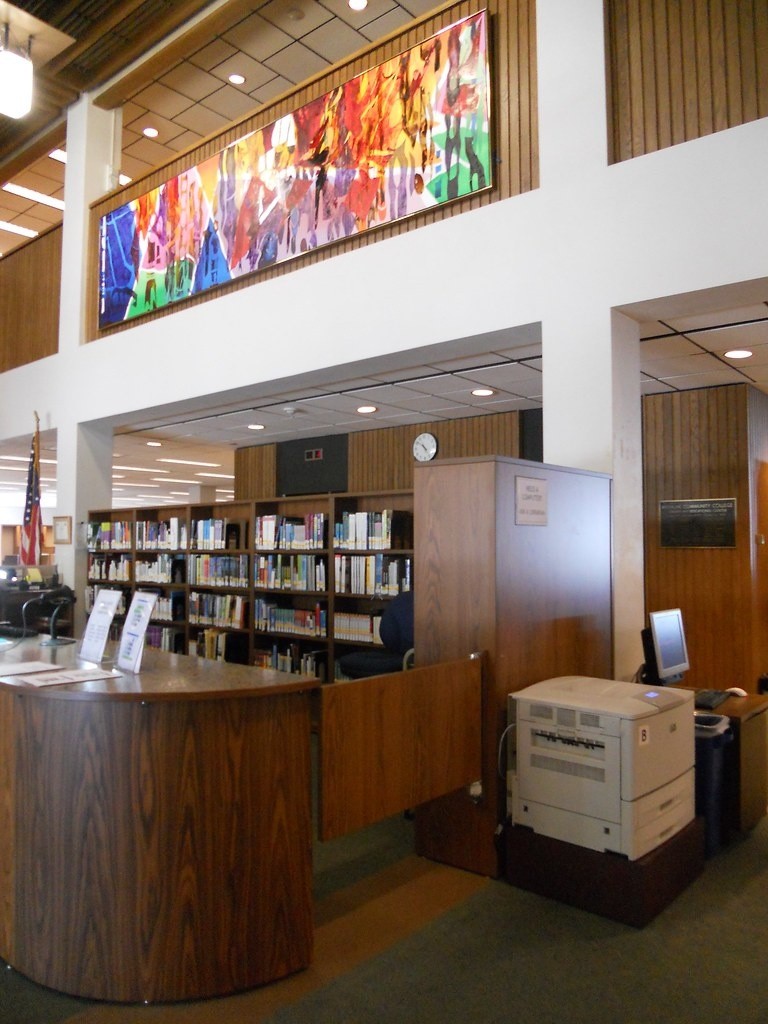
[665,684,768,836]
[87,488,417,736]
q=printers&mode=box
[508,674,696,861]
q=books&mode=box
[85,521,133,640]
[189,516,250,665]
[334,510,414,682]
[253,512,327,682]
[135,517,186,653]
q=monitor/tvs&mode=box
[641,608,692,682]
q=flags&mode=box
[18,432,45,564]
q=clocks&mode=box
[412,432,439,462]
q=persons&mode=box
[339,591,414,679]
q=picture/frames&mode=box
[54,516,73,544]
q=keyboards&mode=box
[694,689,731,710]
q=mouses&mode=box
[724,687,748,697]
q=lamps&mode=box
[1,21,33,120]
